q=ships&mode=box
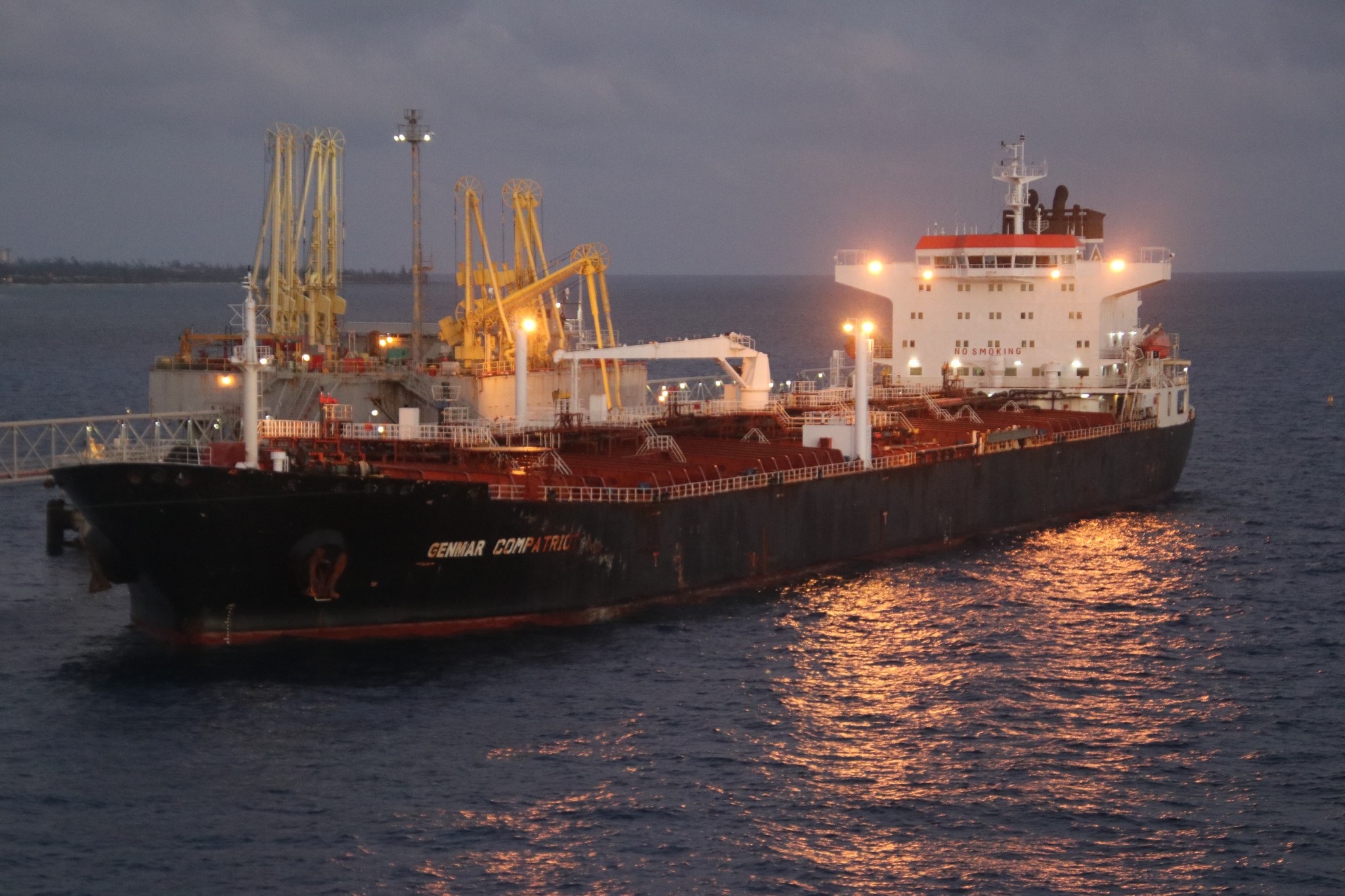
[31,97,1198,666]
